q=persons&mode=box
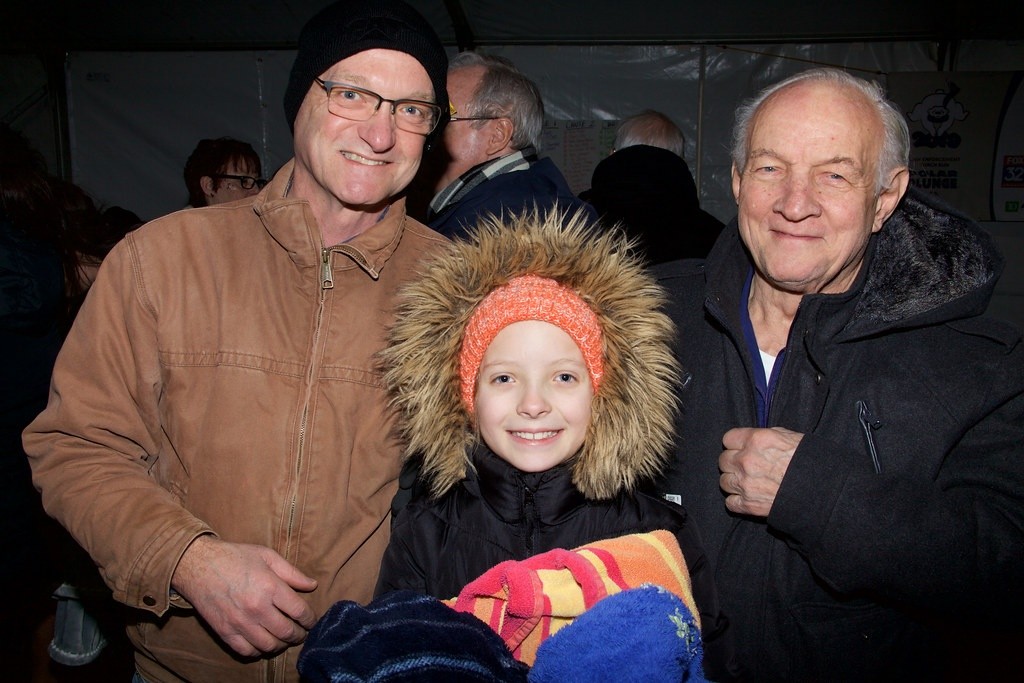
[371,194,755,683]
[408,48,605,249]
[0,120,148,666]
[576,110,727,272]
[183,137,267,209]
[20,1,458,683]
[640,67,1023,683]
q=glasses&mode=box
[215,174,267,190]
[316,75,442,137]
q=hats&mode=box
[284,2,449,140]
[462,274,604,433]
